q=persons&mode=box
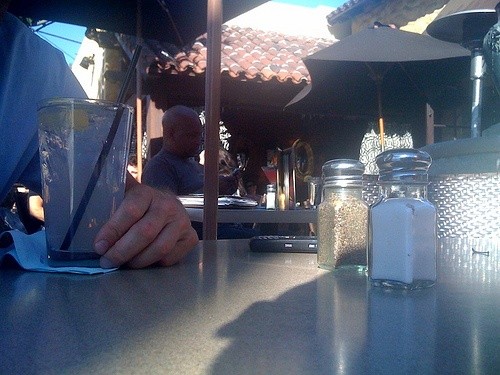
[0,7,199,270]
[140,105,239,197]
[26,188,46,223]
[126,138,292,204]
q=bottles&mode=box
[316,158,369,275]
[367,148,438,289]
[266,184,277,210]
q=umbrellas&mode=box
[280,22,473,153]
[0,0,272,184]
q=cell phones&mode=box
[249,236,318,254]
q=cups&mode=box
[36,97,134,268]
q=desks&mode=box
[183,208,321,225]
[0,239,500,375]
